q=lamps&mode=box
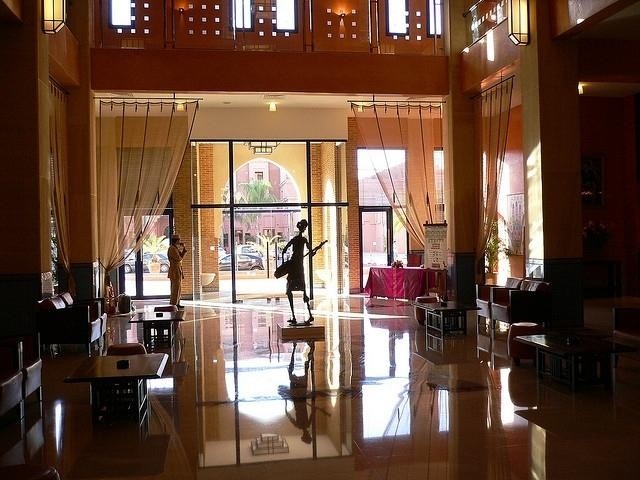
[507,0,529,47]
[41,0,67,37]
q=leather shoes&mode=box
[176,304,184,308]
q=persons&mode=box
[167,234,187,311]
[280,217,326,327]
[284,341,317,445]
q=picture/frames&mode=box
[581,151,607,208]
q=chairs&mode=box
[34,296,105,359]
[0,332,42,420]
[609,305,640,369]
[475,277,551,331]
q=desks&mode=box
[364,268,430,300]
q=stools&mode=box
[415,297,437,326]
[507,322,544,366]
[154,305,179,331]
[106,343,147,355]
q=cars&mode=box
[125,252,170,273]
[218,244,264,270]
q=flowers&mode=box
[584,221,611,246]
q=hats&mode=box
[171,235,180,239]
